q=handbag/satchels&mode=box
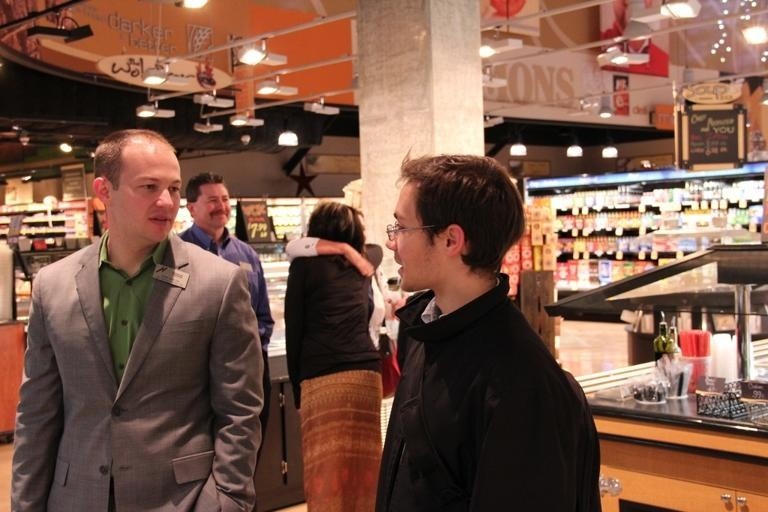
[378,333,401,400]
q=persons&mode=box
[282,200,383,511]
[172,170,275,468]
[373,151,606,512]
[8,127,269,512]
[286,208,406,357]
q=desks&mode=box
[585,367,767,512]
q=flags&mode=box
[596,2,671,80]
[478,0,542,38]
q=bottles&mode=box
[652,320,679,353]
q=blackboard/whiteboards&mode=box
[673,103,746,172]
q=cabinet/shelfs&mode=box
[168,195,345,356]
[499,158,768,320]
[1,197,95,324]
[249,355,304,511]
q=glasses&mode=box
[386,224,442,241]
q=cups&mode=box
[683,355,712,393]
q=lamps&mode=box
[511,144,528,157]
[567,145,584,159]
[135,10,359,146]
[603,147,617,158]
[709,1,767,64]
[598,95,611,118]
[657,0,702,20]
[481,42,494,59]
[610,52,627,63]
[1,117,113,154]
